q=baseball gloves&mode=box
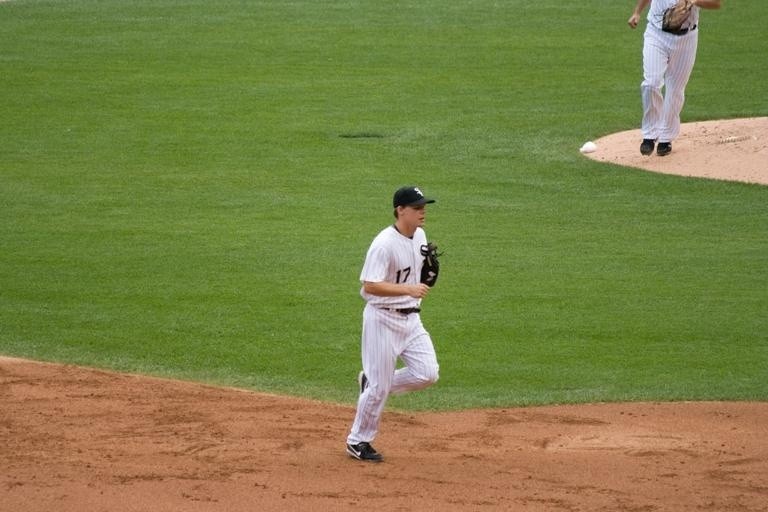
[662,0,699,36]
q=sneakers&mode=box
[345,440,385,462]
[357,370,369,393]
[640,137,673,158]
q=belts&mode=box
[378,307,421,315]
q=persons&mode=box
[345,186,441,463]
[628,0,720,156]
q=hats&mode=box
[391,184,435,208]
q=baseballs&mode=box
[582,141,596,153]
